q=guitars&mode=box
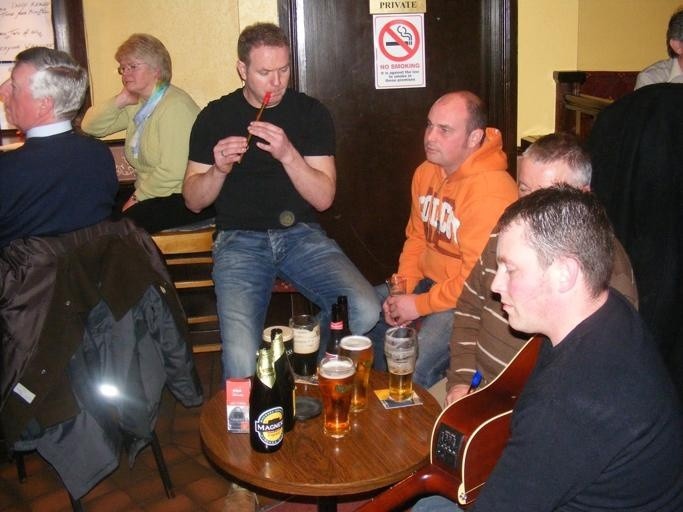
[352,333,545,512]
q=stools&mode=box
[265,278,297,327]
[150,222,221,354]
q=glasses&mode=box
[118,58,153,75]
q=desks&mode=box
[0,129,134,187]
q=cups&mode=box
[386,276,407,327]
[384,325,418,400]
[339,336,374,412]
[321,354,354,438]
[290,314,324,373]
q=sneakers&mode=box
[220,486,259,512]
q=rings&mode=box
[220,149,225,156]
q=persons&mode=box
[80,33,215,236]
[410,180,682,512]
[182,22,382,511]
[0,45,121,248]
[363,89,519,389]
[633,6,682,92]
[443,130,638,408]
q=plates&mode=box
[295,395,320,420]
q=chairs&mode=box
[0,215,202,511]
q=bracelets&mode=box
[129,194,139,203]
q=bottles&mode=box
[326,303,347,357]
[249,346,284,451]
[261,324,294,370]
[338,295,352,337]
[269,329,296,431]
[465,370,482,394]
